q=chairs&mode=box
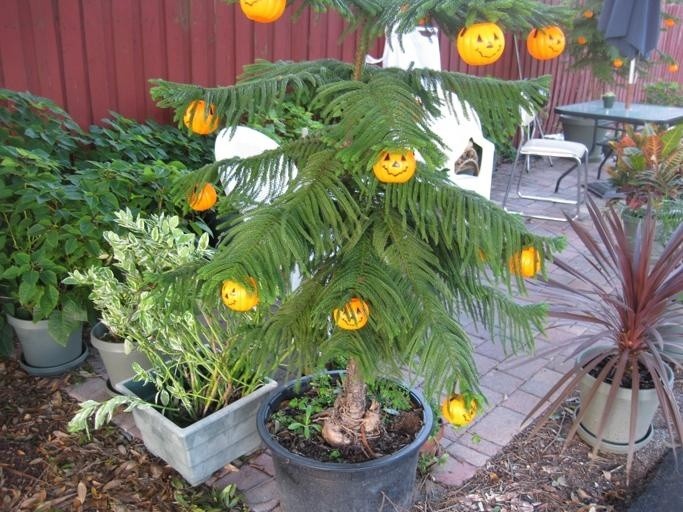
[502,85,593,225]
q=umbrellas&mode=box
[596,1,662,141]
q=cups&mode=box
[602,95,615,108]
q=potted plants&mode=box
[602,92,617,109]
[1,1,565,511]
[496,119,683,488]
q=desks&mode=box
[554,94,683,197]
